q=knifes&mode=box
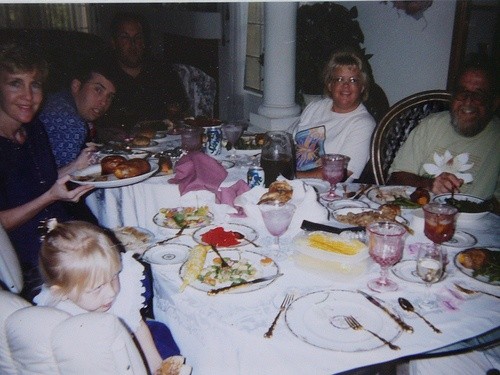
[357,290,414,334]
[207,273,284,296]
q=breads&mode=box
[158,157,171,171]
[459,248,487,270]
[101,156,149,179]
[132,129,157,146]
[410,189,429,205]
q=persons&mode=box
[0,44,180,360]
[99,16,189,127]
[386,55,500,216]
[33,218,163,375]
[37,57,119,228]
[284,53,376,183]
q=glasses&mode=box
[454,88,494,106]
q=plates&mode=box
[111,185,500,353]
[180,117,223,129]
[67,160,160,188]
[299,178,343,196]
[220,160,234,170]
[152,172,175,177]
[221,137,262,155]
[94,123,181,158]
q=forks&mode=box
[210,243,232,270]
[264,293,295,338]
[344,316,401,350]
[453,283,500,299]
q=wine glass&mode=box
[258,201,297,256]
[414,242,444,310]
[321,154,350,201]
[421,201,457,266]
[222,123,243,154]
[366,220,406,293]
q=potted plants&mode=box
[296,2,372,109]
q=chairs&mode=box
[370,90,456,186]
[0,289,149,375]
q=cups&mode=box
[261,130,293,187]
[181,127,202,151]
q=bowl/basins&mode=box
[431,193,490,221]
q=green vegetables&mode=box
[198,257,253,285]
[443,199,489,212]
[471,249,500,283]
[173,213,187,227]
[391,196,420,208]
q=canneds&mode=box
[207,128,222,156]
[246,166,266,188]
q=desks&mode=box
[149,181,500,375]
[86,124,268,228]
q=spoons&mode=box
[398,297,441,335]
[445,183,458,204]
[233,232,259,248]
